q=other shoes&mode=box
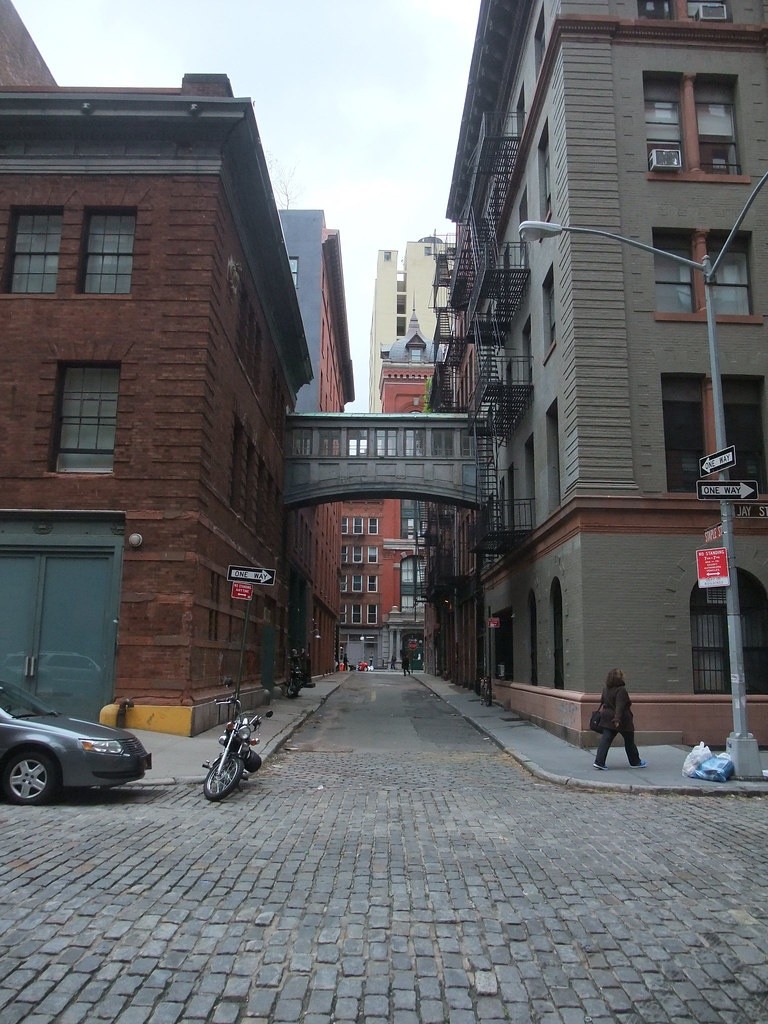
[593,763,608,770]
[630,761,647,768]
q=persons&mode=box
[402,655,410,676]
[290,649,312,683]
[369,652,373,666]
[343,653,349,671]
[593,669,647,770]
[390,655,396,670]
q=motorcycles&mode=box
[202,693,275,802]
[286,648,310,698]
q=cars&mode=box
[3,651,104,693]
[0,680,152,805]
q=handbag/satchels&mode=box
[590,711,604,734]
[682,741,713,777]
[690,753,734,783]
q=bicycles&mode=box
[477,676,492,707]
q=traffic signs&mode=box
[693,480,760,502]
[227,565,277,588]
[698,444,737,479]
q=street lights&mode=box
[515,213,764,779]
